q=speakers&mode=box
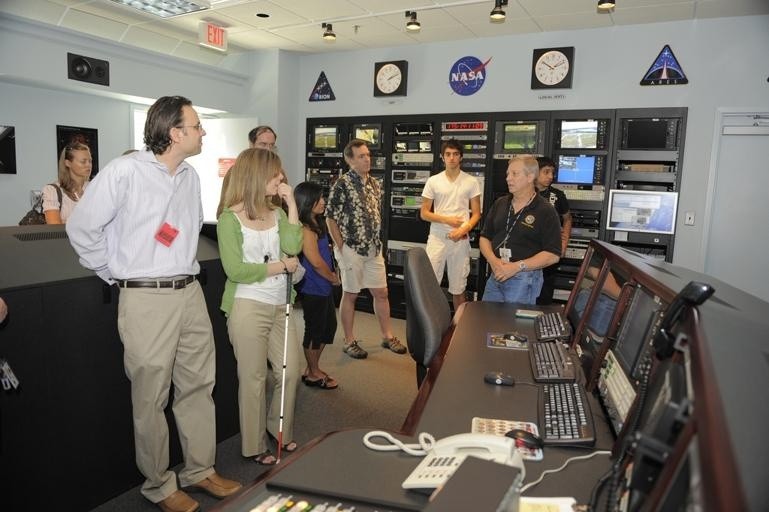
[67,52,109,87]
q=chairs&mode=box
[401,245,454,391]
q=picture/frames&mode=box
[55,124,99,184]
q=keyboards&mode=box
[528,341,576,383]
[534,312,571,342]
[538,383,596,450]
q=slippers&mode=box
[301,372,338,389]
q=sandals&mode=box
[241,449,276,465]
[265,428,297,452]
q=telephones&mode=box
[401,431,526,493]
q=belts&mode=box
[119,275,196,290]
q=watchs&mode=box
[519,259,525,272]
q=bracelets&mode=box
[467,223,473,230]
[280,259,288,274]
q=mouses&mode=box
[505,429,543,449]
[484,371,514,386]
[503,332,527,343]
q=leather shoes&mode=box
[158,490,200,512]
[181,472,243,499]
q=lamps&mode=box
[488,0,511,21]
[597,0,617,10]
[404,10,422,31]
[320,22,338,40]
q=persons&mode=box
[478,155,562,305]
[217,125,288,220]
[324,138,407,359]
[295,181,343,389]
[65,95,243,511]
[216,147,304,465]
[43,141,93,224]
[534,156,572,304]
[420,139,482,312]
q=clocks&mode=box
[373,61,410,98]
[530,47,576,91]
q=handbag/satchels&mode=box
[19,209,47,225]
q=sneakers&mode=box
[381,336,406,354]
[342,340,368,359]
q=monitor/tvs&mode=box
[555,119,608,150]
[606,189,678,235]
[567,249,605,332]
[352,123,382,150]
[622,118,678,151]
[494,120,546,154]
[580,262,630,362]
[553,155,606,184]
[597,283,670,440]
[312,124,339,152]
